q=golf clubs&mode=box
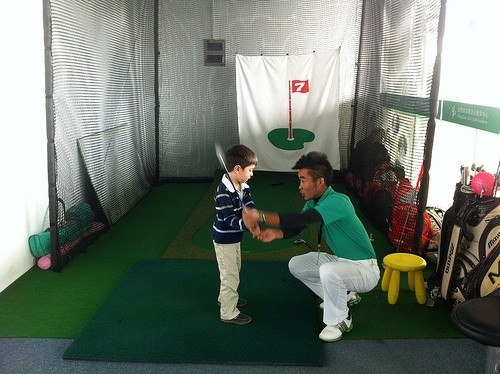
[381,162,425,270]
[460,163,487,187]
[294,238,315,252]
[213,139,248,214]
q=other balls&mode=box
[471,172,495,196]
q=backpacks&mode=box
[350,138,447,263]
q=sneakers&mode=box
[217,295,246,306]
[220,312,251,325]
[320,291,361,309]
[319,314,353,342]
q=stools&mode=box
[381,253,428,306]
[452,297,500,374]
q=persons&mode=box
[245,152,380,344]
[213,145,260,325]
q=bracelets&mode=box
[259,212,266,225]
